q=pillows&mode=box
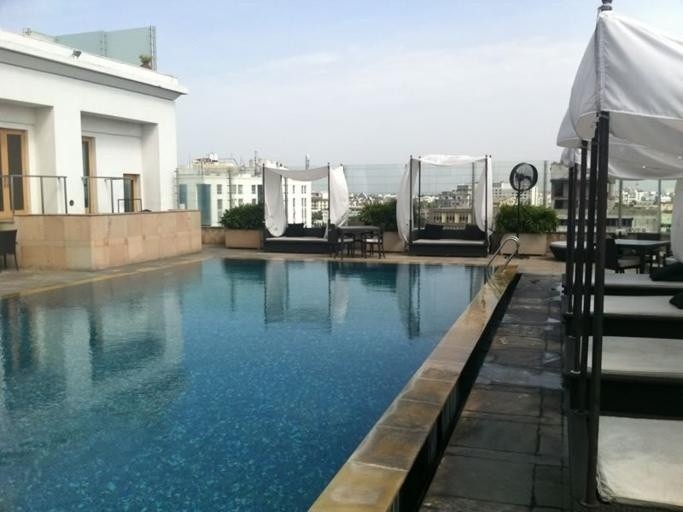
[286,222,304,238]
[424,221,444,240]
[321,223,326,237]
[462,224,482,240]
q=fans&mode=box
[504,163,541,260]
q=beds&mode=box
[262,160,349,253]
[409,153,494,256]
[535,0,681,510]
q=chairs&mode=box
[0,228,21,272]
[329,220,389,259]
